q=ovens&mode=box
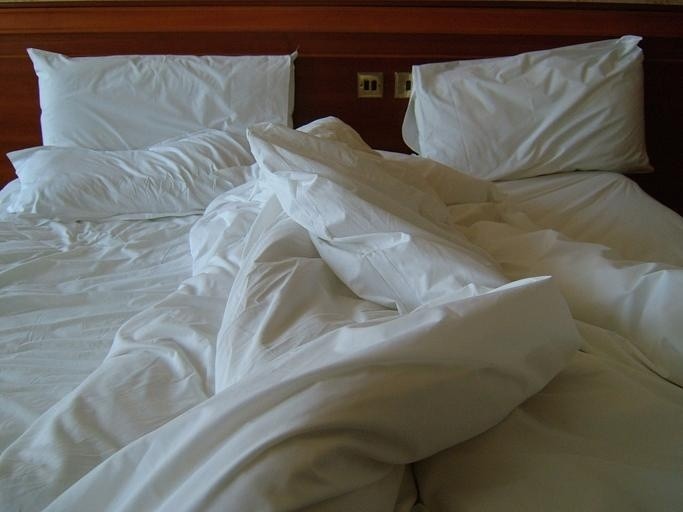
[0,28,683,512]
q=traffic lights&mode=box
[402,33,655,182]
[25,47,300,148]
